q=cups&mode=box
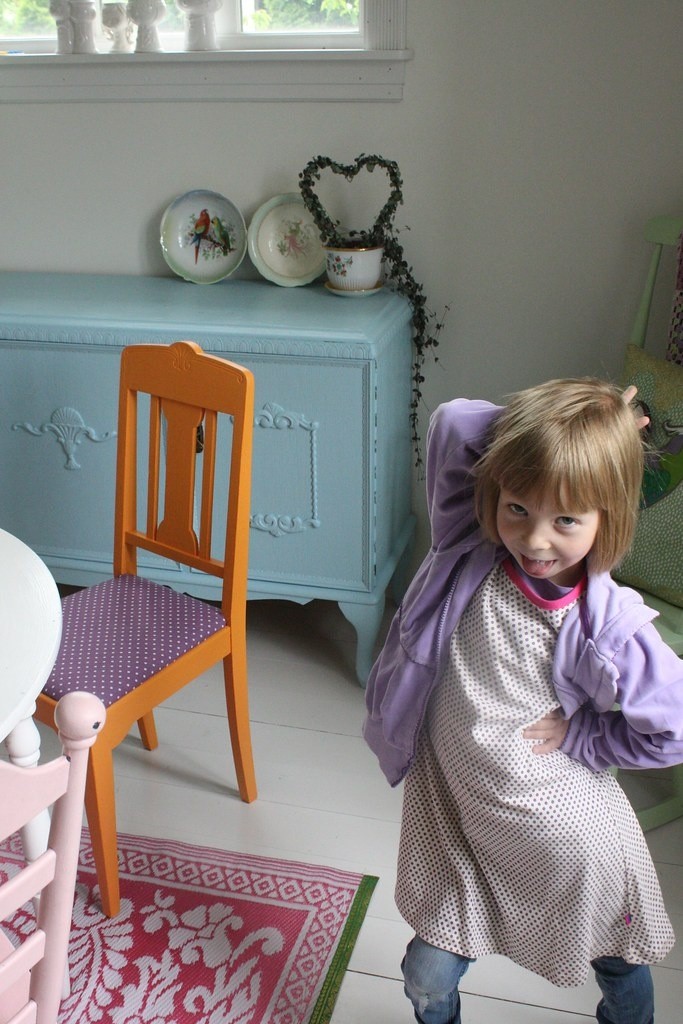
[325,245,385,288]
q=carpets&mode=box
[0,824,380,1024]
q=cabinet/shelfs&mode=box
[0,270,420,690]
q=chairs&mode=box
[606,213,683,831]
[0,691,106,1024]
[32,339,258,918]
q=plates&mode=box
[247,193,327,286]
[160,189,248,284]
[324,282,384,298]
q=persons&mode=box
[362,380,683,1024]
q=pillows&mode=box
[609,343,683,611]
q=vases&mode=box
[49,0,224,54]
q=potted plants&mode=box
[299,153,453,483]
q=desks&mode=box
[0,528,63,923]
